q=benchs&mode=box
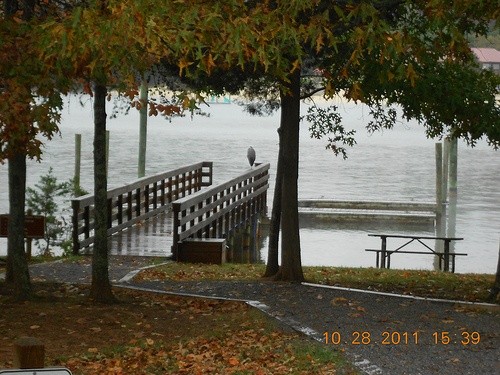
[365,248,467,275]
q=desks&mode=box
[366,233,463,272]
